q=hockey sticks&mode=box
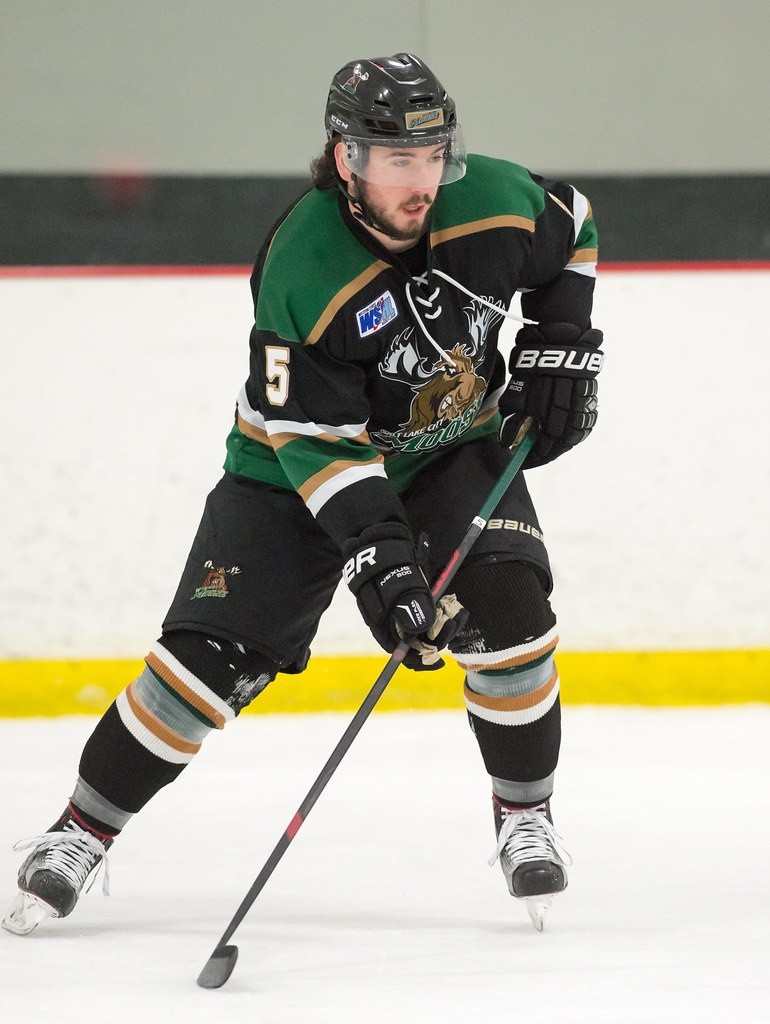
[194,419,544,989]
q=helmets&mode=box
[324,53,457,149]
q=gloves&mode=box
[339,521,470,673]
[497,320,604,464]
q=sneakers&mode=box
[1,801,115,937]
[492,793,572,933]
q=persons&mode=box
[0,53,604,937]
